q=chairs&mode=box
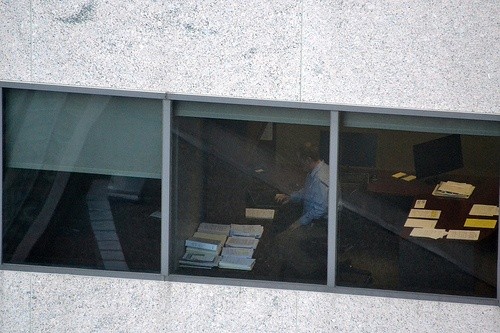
[286,211,344,283]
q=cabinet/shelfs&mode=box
[206,118,277,222]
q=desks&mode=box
[247,189,292,225]
[177,227,271,279]
[398,196,498,297]
[369,171,500,224]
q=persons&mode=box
[276,142,343,284]
